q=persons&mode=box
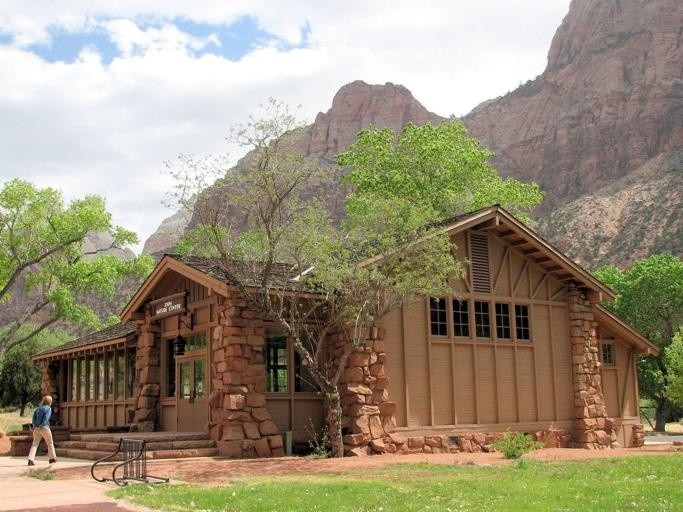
[27,395,58,465]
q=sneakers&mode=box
[49,459,56,463]
[29,461,34,466]
